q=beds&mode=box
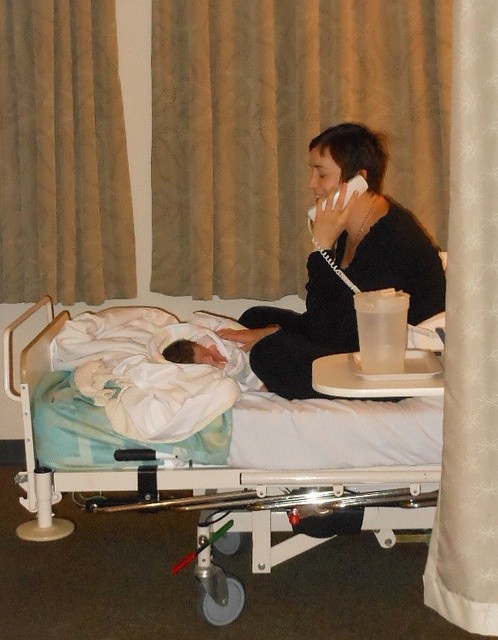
[3,289,444,543]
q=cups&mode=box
[353,288,411,375]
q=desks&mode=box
[312,349,443,399]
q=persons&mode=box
[163,339,231,375]
[216,122,446,402]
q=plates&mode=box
[348,347,444,382]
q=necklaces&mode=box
[347,194,380,244]
